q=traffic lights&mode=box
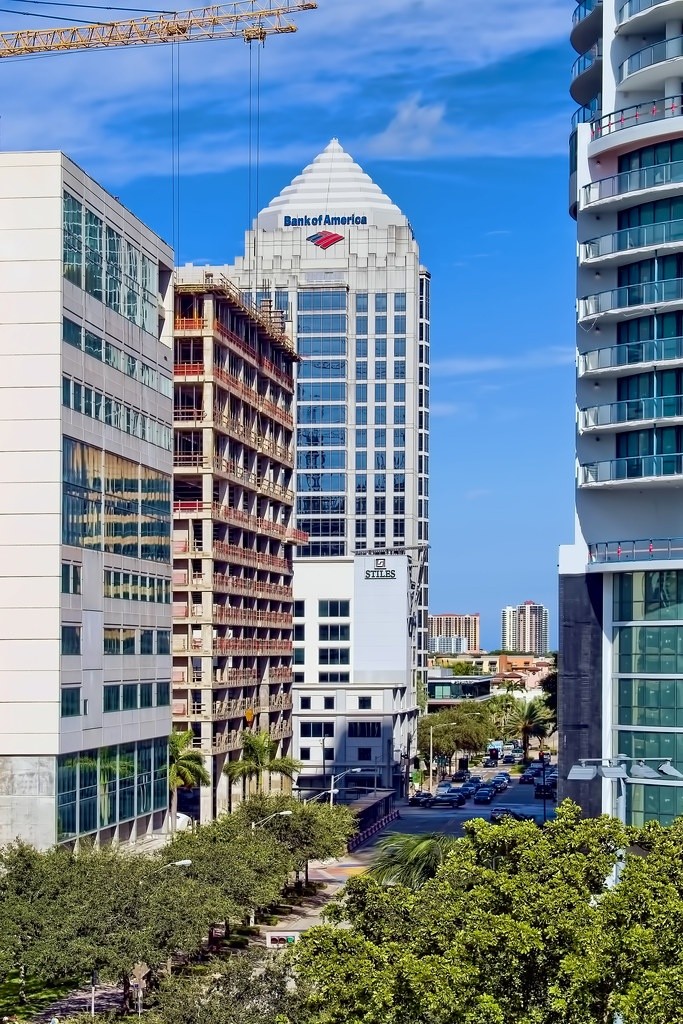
[266,932,300,948]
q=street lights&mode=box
[430,723,456,790]
[303,789,339,808]
[251,811,293,840]
[330,768,361,809]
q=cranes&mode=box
[0,0,318,58]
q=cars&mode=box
[447,774,483,799]
[452,770,471,782]
[535,772,558,799]
[436,783,451,795]
[503,743,524,764]
[478,772,510,800]
[524,751,551,776]
[474,791,491,804]
[483,759,497,767]
[409,793,432,807]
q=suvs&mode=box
[519,774,534,783]
[420,793,466,808]
[491,807,508,821]
